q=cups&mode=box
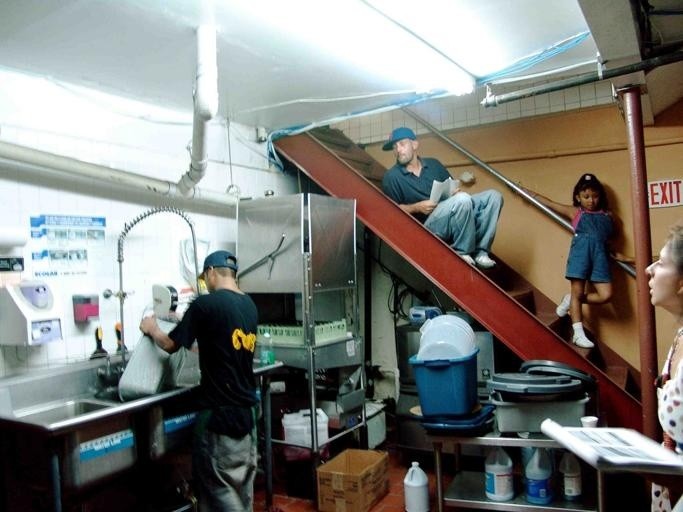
[579,415,599,428]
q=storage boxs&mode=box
[490,393,591,432]
[119,318,189,401]
[62,430,138,487]
[316,448,391,511]
[320,398,386,447]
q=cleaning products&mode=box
[524,447,554,505]
[484,446,515,502]
[558,449,583,502]
[404,462,430,512]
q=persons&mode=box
[644,220,683,512]
[379,127,503,268]
[138,250,258,512]
[510,173,616,348]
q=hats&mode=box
[198,251,239,279]
[381,128,415,151]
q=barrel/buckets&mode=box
[416,313,474,360]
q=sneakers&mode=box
[461,254,475,264]
[476,250,497,268]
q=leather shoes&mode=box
[556,293,571,316]
[574,334,594,348]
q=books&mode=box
[540,418,683,476]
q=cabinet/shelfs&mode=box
[425,432,604,512]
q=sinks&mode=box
[13,398,111,438]
[89,383,182,405]
[250,357,284,373]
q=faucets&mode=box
[118,205,200,375]
